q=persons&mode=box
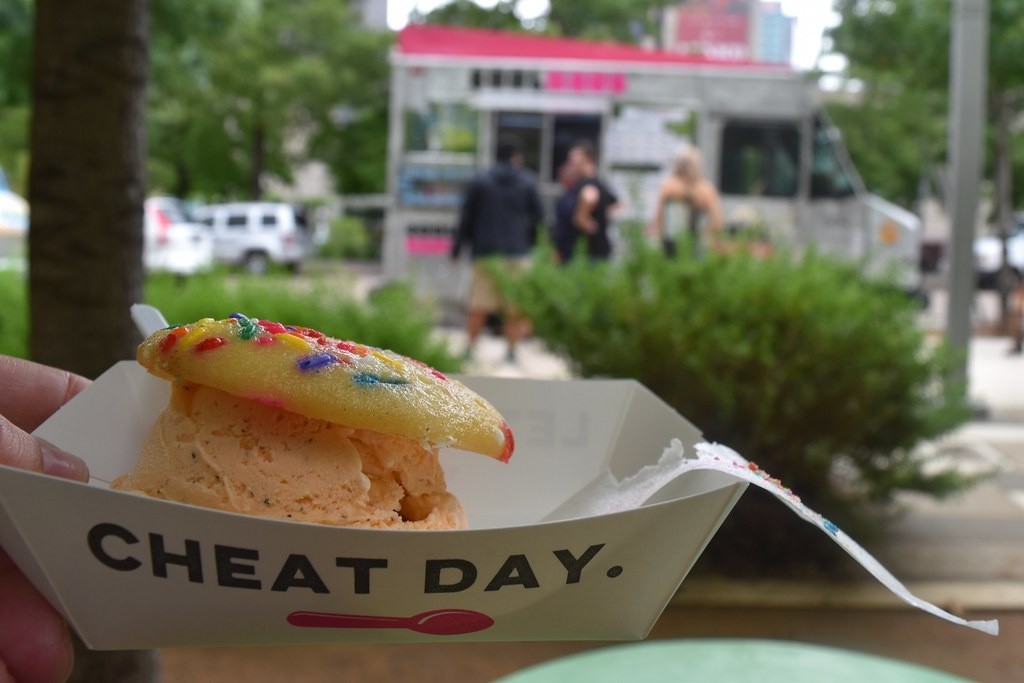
[0,353,95,683]
[446,139,720,365]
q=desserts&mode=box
[105,310,515,532]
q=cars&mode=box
[974,204,1024,293]
[192,199,308,279]
[143,191,209,278]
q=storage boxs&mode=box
[0,358,749,652]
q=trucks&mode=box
[379,19,929,302]
